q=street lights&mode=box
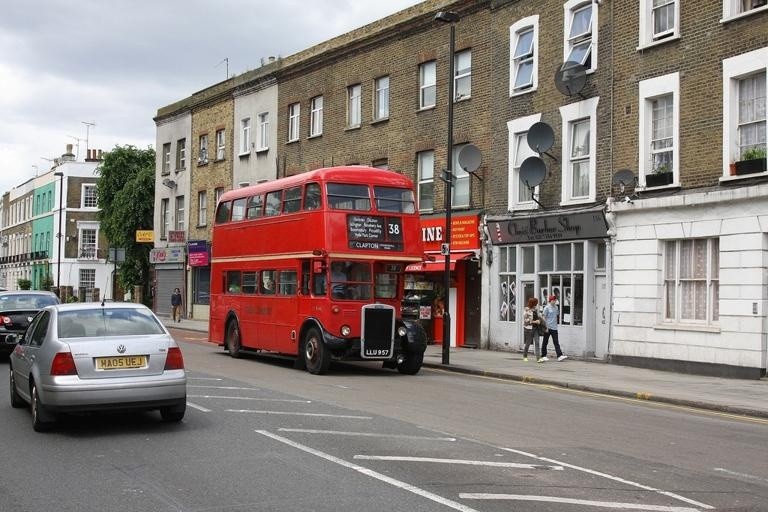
[55,172,63,295]
[435,10,459,366]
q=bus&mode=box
[210,167,428,375]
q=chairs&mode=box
[34,298,49,309]
[2,300,16,310]
[96,313,146,335]
[59,318,85,337]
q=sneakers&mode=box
[523,355,568,363]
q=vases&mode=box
[730,164,737,175]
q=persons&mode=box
[541,289,548,305]
[564,288,570,307]
[324,262,348,299]
[540,295,568,362]
[551,288,560,304]
[171,289,182,323]
[523,298,546,363]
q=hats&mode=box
[548,295,557,302]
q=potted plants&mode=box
[736,143,766,174]
[644,166,673,186]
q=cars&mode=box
[9,301,187,431]
[0,290,60,362]
